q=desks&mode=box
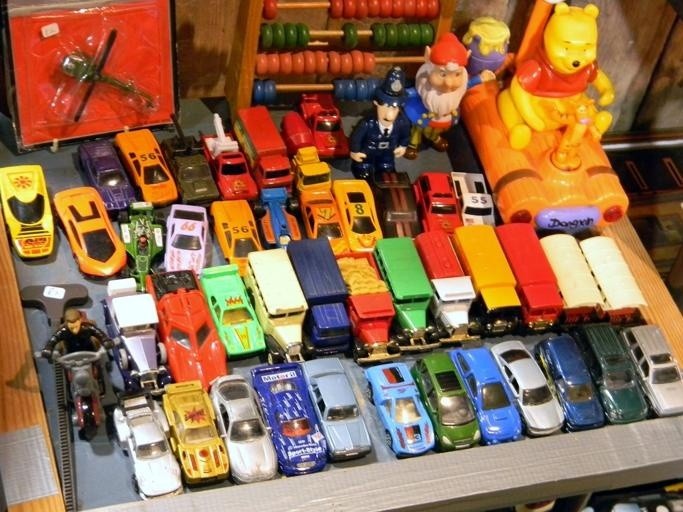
[0,95,683,512]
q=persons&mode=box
[41,308,115,360]
[350,65,411,183]
[415,32,469,153]
